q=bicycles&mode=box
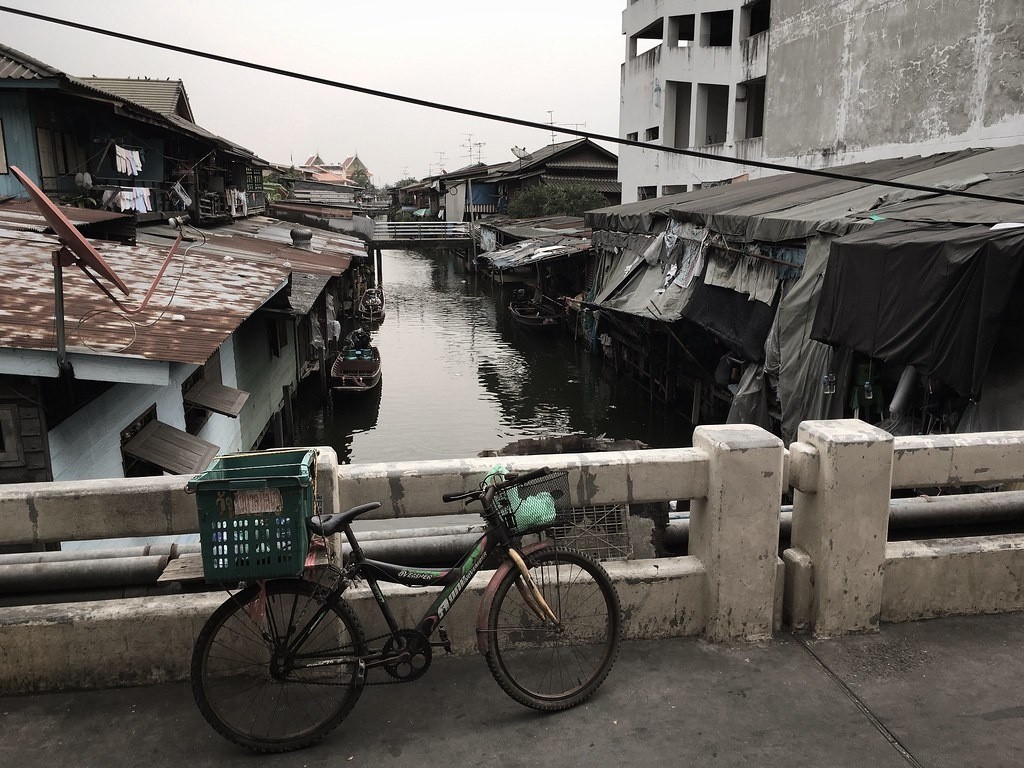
[190,467,623,758]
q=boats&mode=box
[358,288,386,323]
[509,297,560,329]
[328,330,385,397]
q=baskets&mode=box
[492,470,571,529]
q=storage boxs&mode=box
[185,449,320,584]
